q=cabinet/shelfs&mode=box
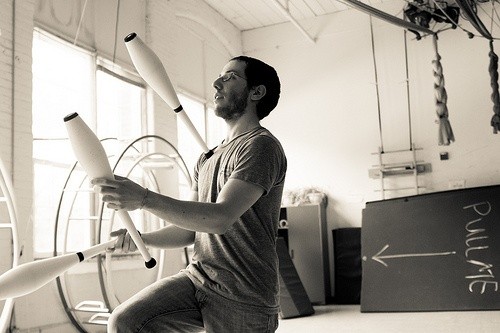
[279,204,331,305]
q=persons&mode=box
[91,55,287,333]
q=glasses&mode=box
[219,70,248,85]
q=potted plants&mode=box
[290,188,324,205]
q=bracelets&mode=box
[138,188,148,209]
